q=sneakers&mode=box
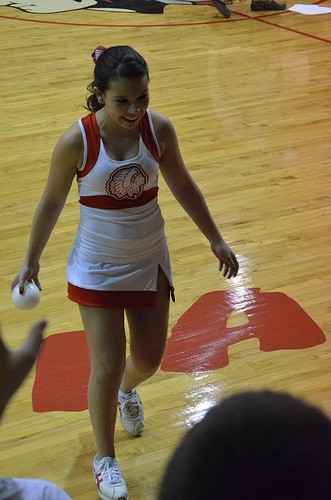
[118,388,144,437]
[92,453,129,500]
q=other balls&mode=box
[12,282,41,310]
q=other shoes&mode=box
[250,1,286,10]
